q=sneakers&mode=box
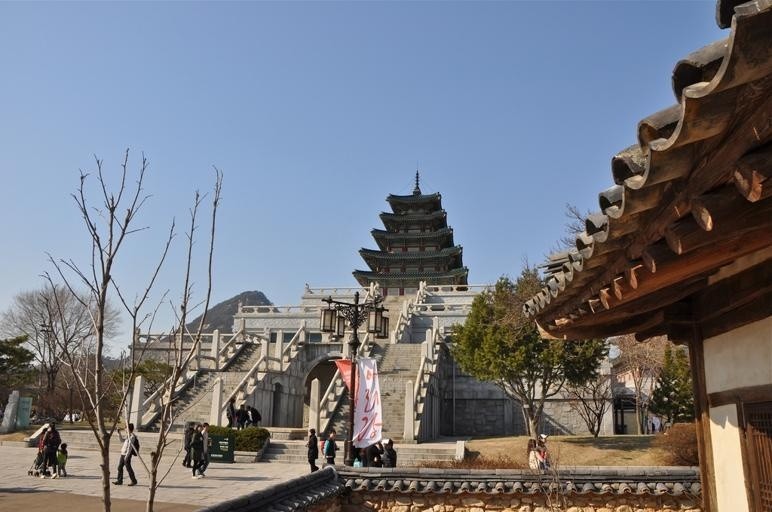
[128,481,137,486]
[51,473,57,479]
[112,481,122,485]
[191,468,204,479]
[41,475,46,479]
[184,461,193,467]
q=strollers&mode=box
[27,445,53,478]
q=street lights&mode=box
[319,289,390,466]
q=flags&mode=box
[333,358,383,449]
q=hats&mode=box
[538,433,549,439]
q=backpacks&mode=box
[321,437,332,455]
[131,435,139,456]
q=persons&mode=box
[39,427,48,453]
[226,398,262,430]
[321,429,339,464]
[190,424,204,479]
[353,438,397,468]
[197,422,212,477]
[112,422,138,486]
[39,421,62,479]
[526,438,546,470]
[532,434,551,469]
[181,421,195,468]
[304,428,319,472]
[56,443,68,477]
